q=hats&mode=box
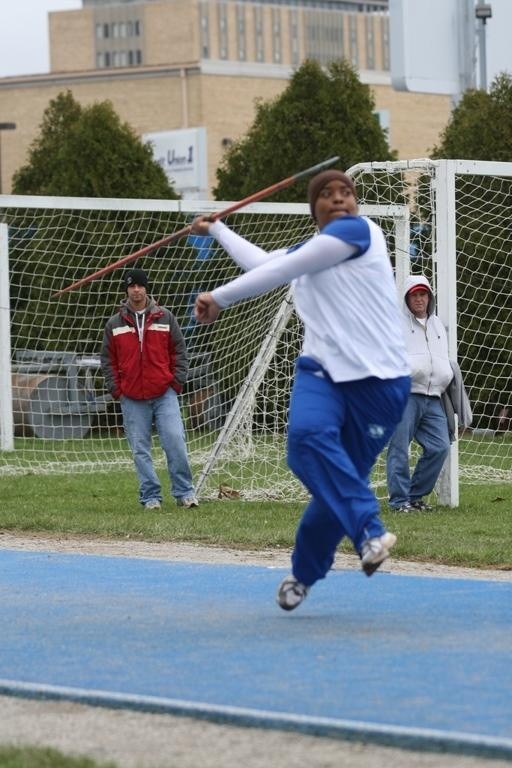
[408,285,429,295]
[308,170,357,221]
[124,270,149,291]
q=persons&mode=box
[189,169,413,610]
[101,269,198,510]
[387,276,453,513]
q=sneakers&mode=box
[278,574,309,610]
[178,497,197,508]
[361,532,396,576]
[396,498,431,515]
[145,500,162,512]
